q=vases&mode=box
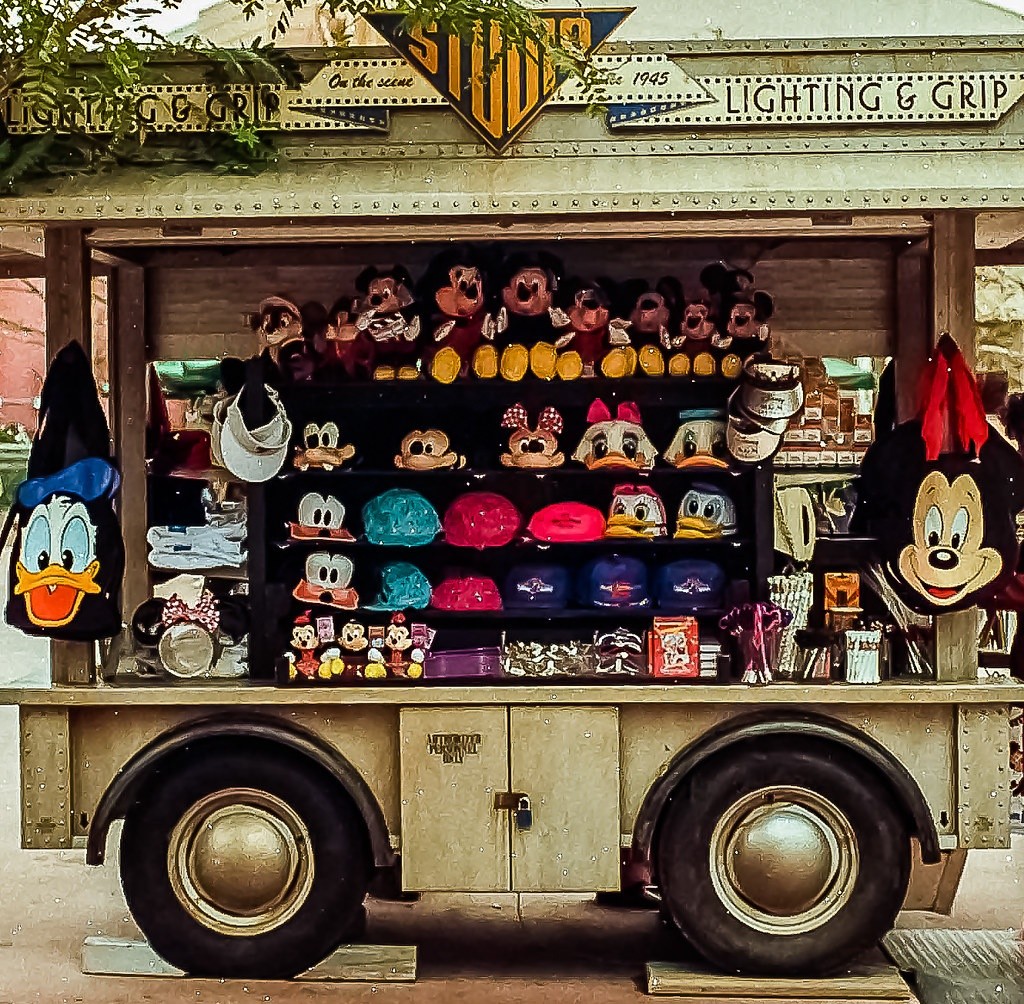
[0,443,31,510]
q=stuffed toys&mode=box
[221,250,774,383]
[284,610,436,679]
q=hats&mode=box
[443,492,523,550]
[657,401,734,475]
[723,347,804,469]
[670,480,742,540]
[657,559,725,611]
[212,384,294,484]
[491,398,570,478]
[587,552,652,609]
[500,565,569,609]
[360,489,443,548]
[361,563,432,611]
[431,575,502,612]
[291,412,356,475]
[274,489,358,550]
[390,415,471,480]
[291,550,360,611]
[527,502,607,545]
[603,484,670,540]
[571,394,661,477]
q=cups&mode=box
[899,626,934,679]
[738,630,783,686]
[846,631,882,684]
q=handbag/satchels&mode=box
[853,333,1024,615]
[0,338,127,641]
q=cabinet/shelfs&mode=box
[244,350,777,685]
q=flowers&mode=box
[0,421,31,445]
